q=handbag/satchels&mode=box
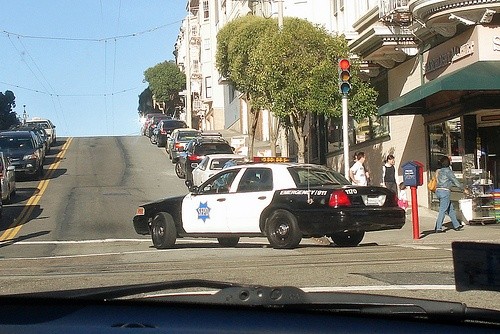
[428,177,437,193]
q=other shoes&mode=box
[455,225,464,231]
[435,229,442,233]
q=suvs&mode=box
[0,117,56,214]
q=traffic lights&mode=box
[338,57,352,94]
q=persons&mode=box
[435,156,470,233]
[398,182,408,213]
[349,151,369,186]
[382,155,398,197]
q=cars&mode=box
[133,156,406,249]
[140,114,261,192]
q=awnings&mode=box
[378,61,500,116]
